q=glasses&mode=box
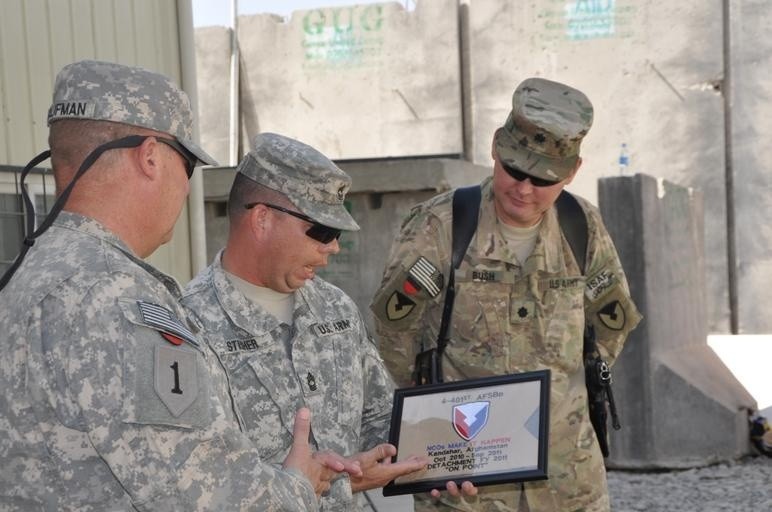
[141,135,197,179]
[501,160,559,187]
[244,202,342,244]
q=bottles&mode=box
[619,142,632,177]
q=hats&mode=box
[235,132,361,232]
[46,59,219,169]
[494,77,594,183]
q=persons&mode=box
[0,56,369,511]
[179,130,484,510]
[359,73,648,510]
